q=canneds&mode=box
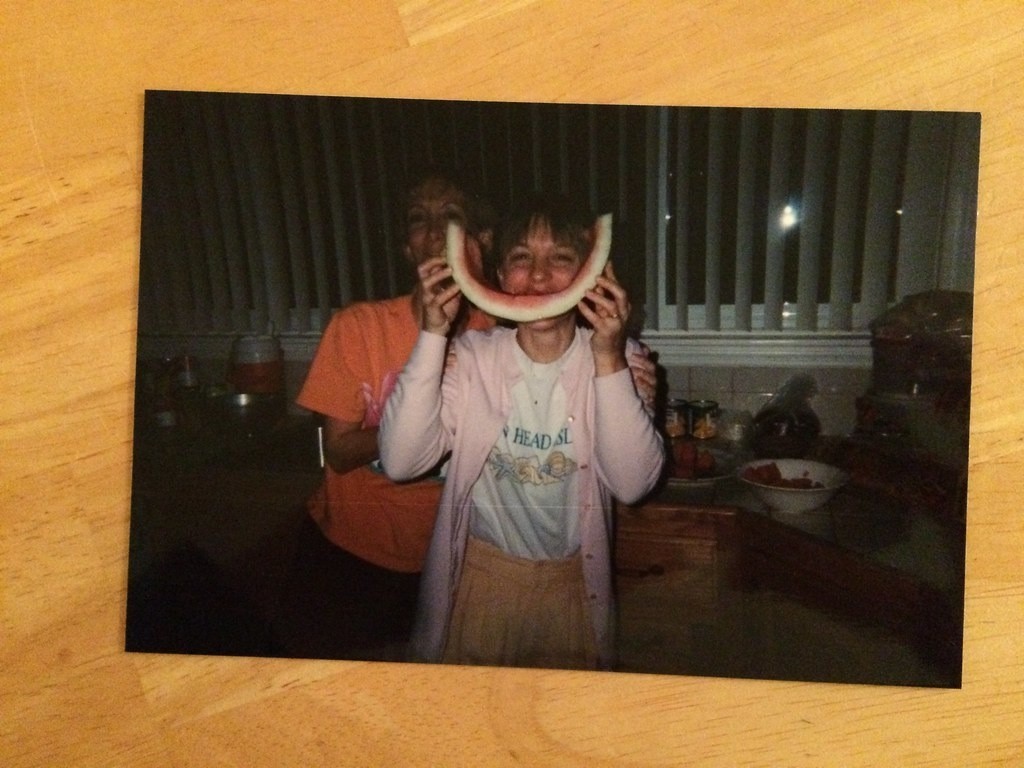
[658,398,719,440]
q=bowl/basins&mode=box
[734,459,850,516]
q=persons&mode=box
[376,195,668,670]
[268,162,660,664]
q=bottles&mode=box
[170,356,199,400]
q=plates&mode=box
[659,448,740,487]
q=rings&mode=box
[608,311,621,319]
